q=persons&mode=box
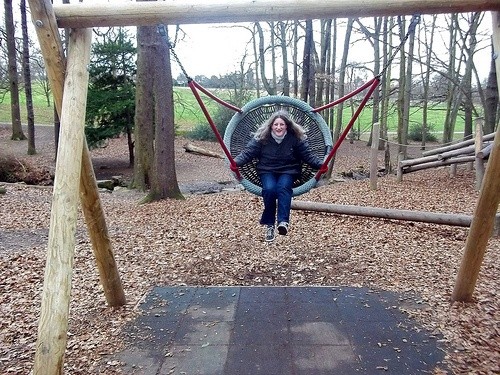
[230,111,330,243]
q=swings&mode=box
[156,15,422,197]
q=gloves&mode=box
[319,164,328,174]
[230,161,238,172]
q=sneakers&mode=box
[277,222,289,235]
[265,225,275,240]
